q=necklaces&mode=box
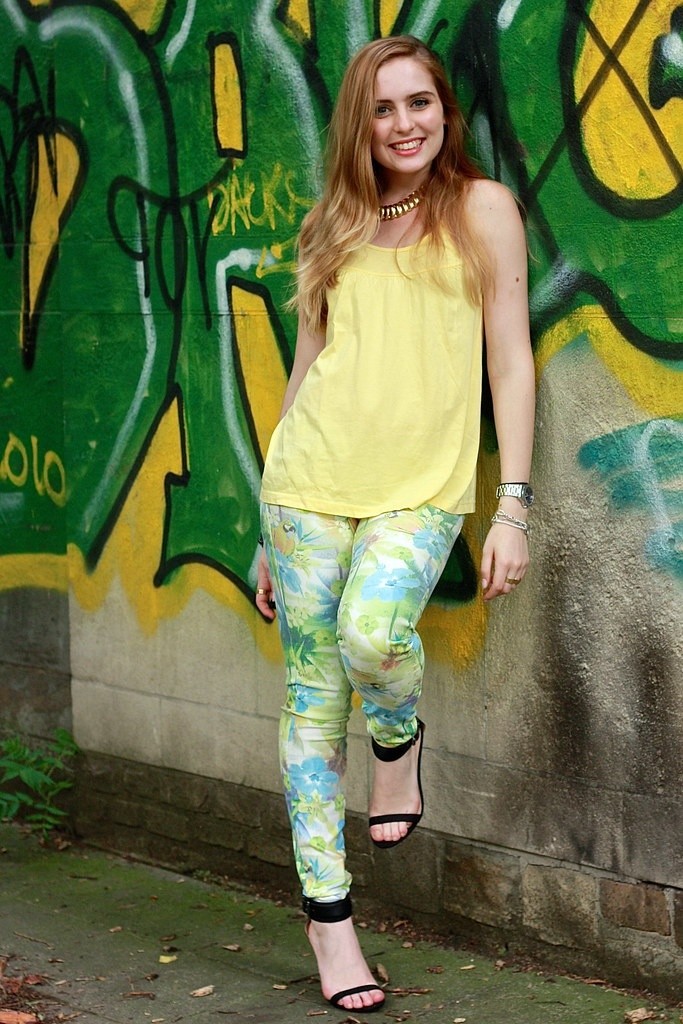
[378,183,426,221]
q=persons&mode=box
[254,33,535,1010]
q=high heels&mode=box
[367,717,426,848]
[299,895,385,1013]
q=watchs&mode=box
[495,482,534,508]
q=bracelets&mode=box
[490,510,530,535]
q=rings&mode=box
[505,577,514,584]
[256,588,266,594]
[515,578,522,586]
